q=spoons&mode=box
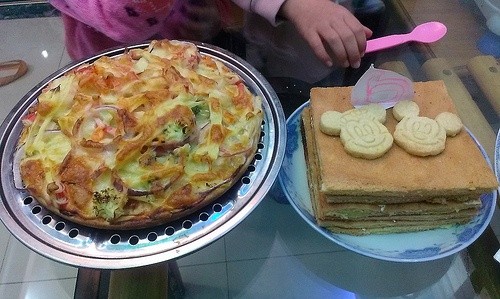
[357,21,447,52]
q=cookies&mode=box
[319,102,394,159]
[392,99,462,157]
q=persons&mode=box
[49,0,244,62]
[233,0,373,113]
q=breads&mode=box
[301,79,499,235]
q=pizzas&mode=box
[19,38,263,232]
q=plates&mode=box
[277,96,500,262]
